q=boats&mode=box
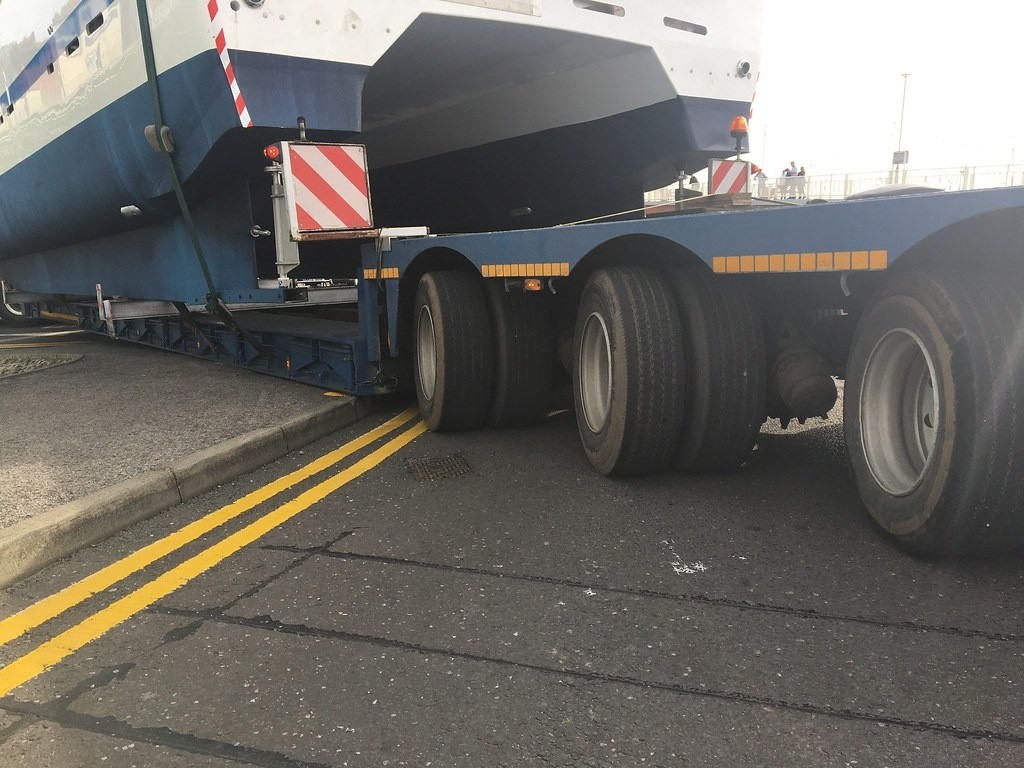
[0,0,764,306]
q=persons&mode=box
[754,169,767,197]
[689,175,700,191]
[778,161,806,199]
